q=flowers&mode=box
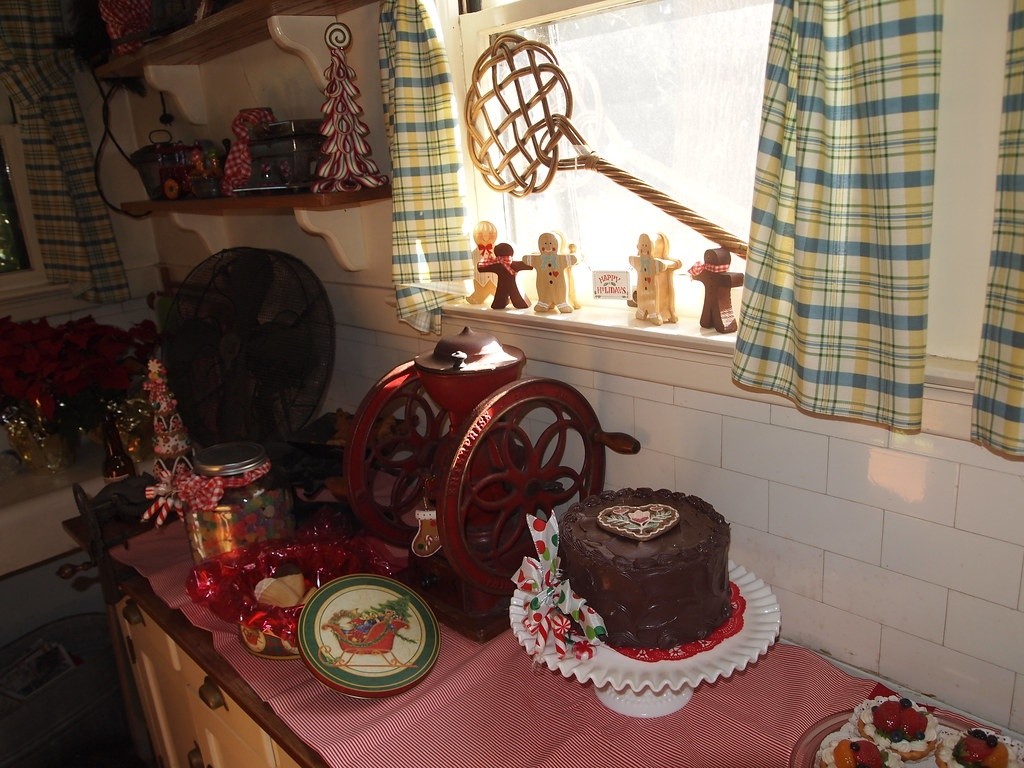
[0,315,159,433]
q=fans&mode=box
[161,247,335,447]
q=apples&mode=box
[164,179,180,199]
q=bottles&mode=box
[1,406,42,464]
[102,420,136,487]
[186,440,296,568]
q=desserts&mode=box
[818,698,1017,768]
[252,565,319,608]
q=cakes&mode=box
[555,487,732,650]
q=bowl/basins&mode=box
[188,176,221,199]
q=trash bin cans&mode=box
[0,612,138,768]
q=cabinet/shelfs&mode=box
[116,596,301,768]
[95,0,392,216]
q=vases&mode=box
[98,411,136,486]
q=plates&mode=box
[790,708,977,768]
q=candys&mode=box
[183,483,294,566]
